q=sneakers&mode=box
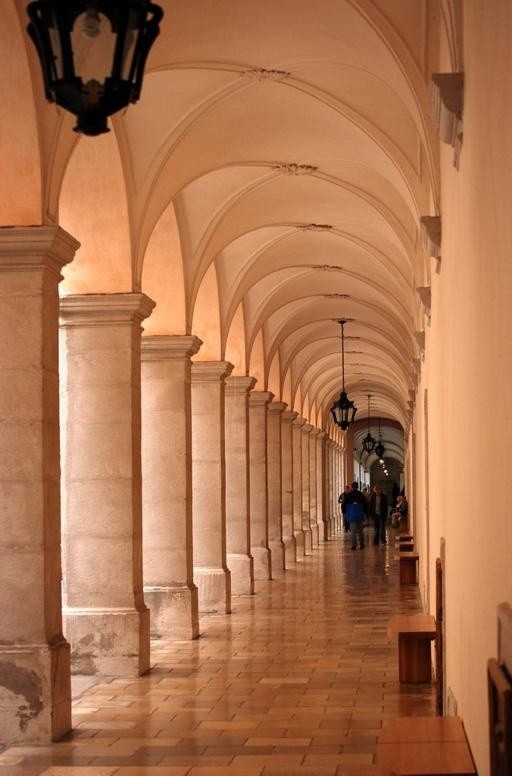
[360,544,366,550]
[350,545,357,550]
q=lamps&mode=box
[328,312,362,429]
[362,390,386,456]
[22,0,174,138]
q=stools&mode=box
[378,715,479,776]
[394,517,419,586]
[385,611,439,684]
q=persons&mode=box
[338,482,407,550]
